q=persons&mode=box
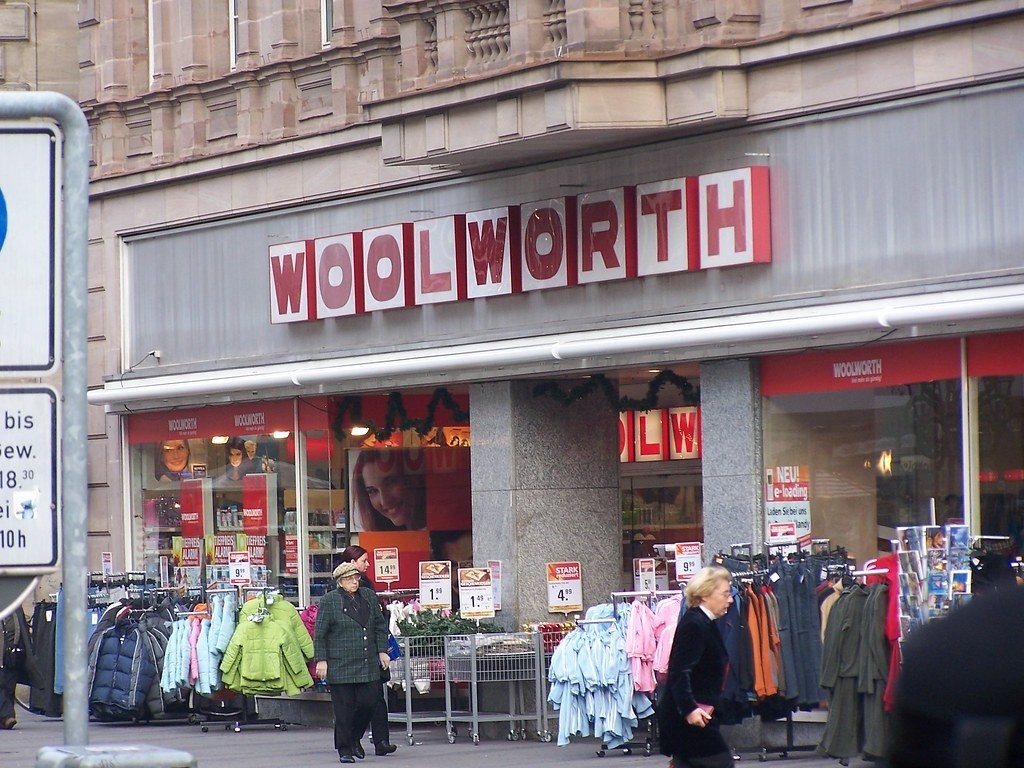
[0,604,37,730]
[657,567,734,768]
[429,529,473,610]
[351,448,427,532]
[343,546,397,756]
[154,439,191,484]
[226,436,273,482]
[313,563,392,763]
[888,574,1024,768]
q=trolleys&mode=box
[369,630,575,745]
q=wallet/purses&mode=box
[687,703,714,723]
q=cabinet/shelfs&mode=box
[143,526,346,578]
[369,629,575,747]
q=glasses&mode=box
[342,575,361,581]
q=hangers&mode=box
[827,569,890,588]
[128,608,157,624]
[732,569,771,596]
[158,586,202,611]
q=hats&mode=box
[969,549,1017,594]
[333,563,359,581]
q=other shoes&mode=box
[0,717,17,729]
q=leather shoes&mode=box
[340,754,355,763]
[374,741,396,755]
[352,743,365,759]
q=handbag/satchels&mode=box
[4,645,26,669]
[379,666,390,683]
